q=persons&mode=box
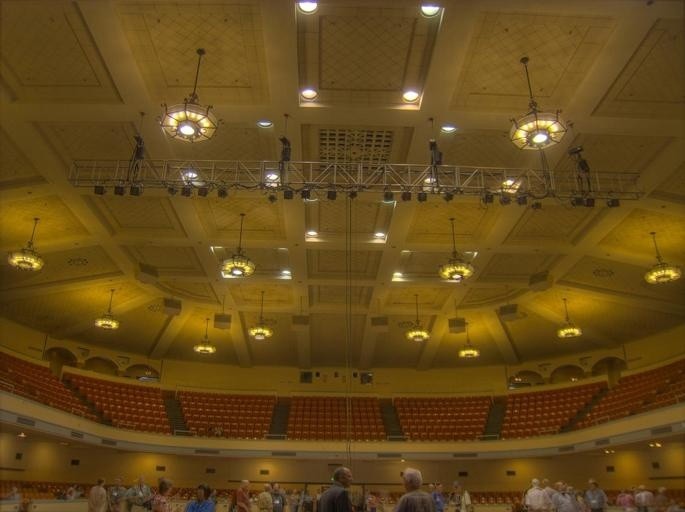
[360,489,386,510]
[397,468,438,511]
[427,479,472,511]
[317,467,354,511]
[87,478,321,512]
[511,478,675,511]
[8,481,85,511]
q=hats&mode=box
[402,467,423,487]
[531,478,573,493]
